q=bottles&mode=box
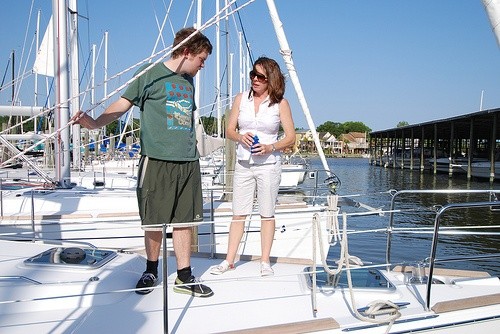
[251,136,261,154]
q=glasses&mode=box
[250,70,268,83]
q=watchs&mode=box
[270,144,276,153]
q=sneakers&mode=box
[135,271,159,294]
[259,262,273,276]
[210,259,235,275]
[173,276,214,297]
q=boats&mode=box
[0,0,500,334]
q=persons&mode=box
[210,58,296,277]
[68,26,213,298]
